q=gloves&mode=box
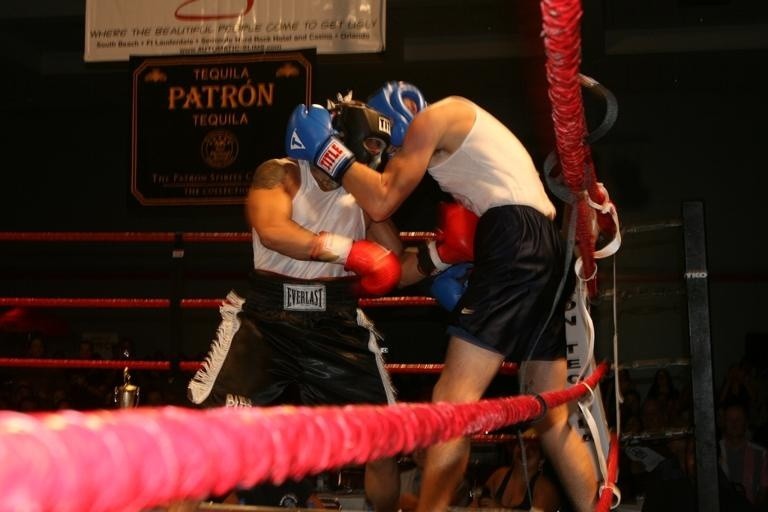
[417,199,480,278]
[311,232,401,295]
[284,103,356,185]
[430,263,474,311]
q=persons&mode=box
[168,86,479,512]
[282,76,597,512]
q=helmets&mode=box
[333,79,427,169]
